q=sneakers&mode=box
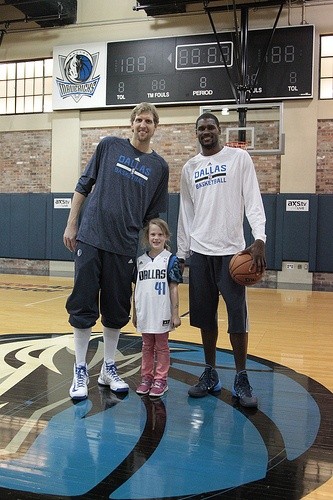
[149,379,169,396]
[136,381,153,394]
[97,361,129,392]
[69,363,90,400]
[232,370,258,408]
[188,364,221,398]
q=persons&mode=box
[60,99,171,401]
[130,217,183,396]
[173,112,267,411]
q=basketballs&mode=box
[229,252,263,285]
